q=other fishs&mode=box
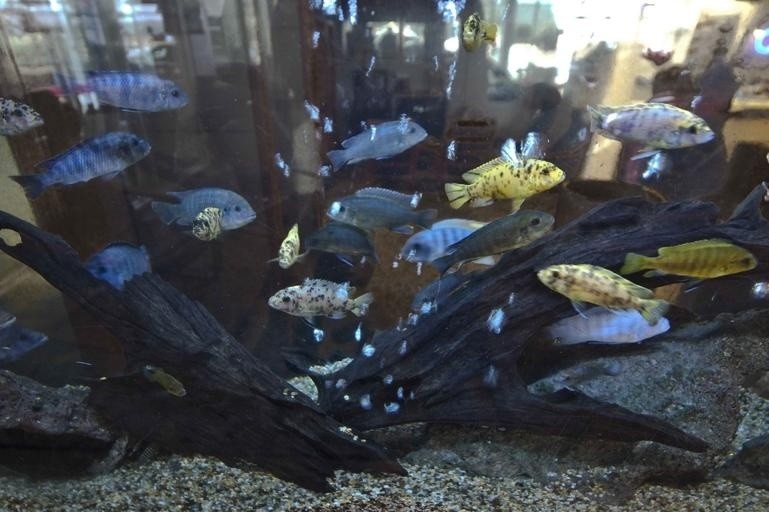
[268,116,566,329]
